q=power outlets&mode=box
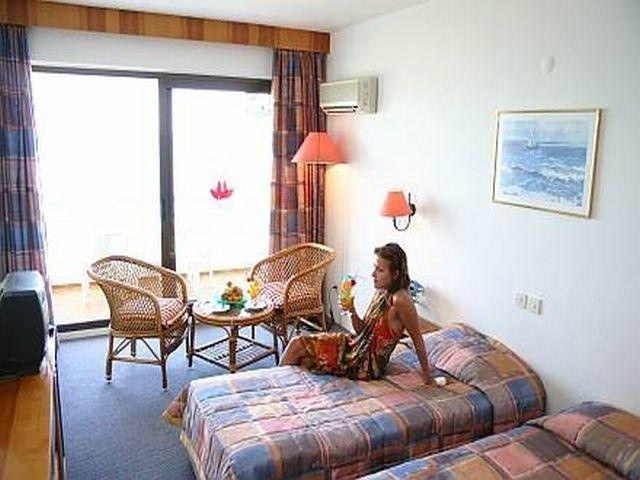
[329,280,340,294]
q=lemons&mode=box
[341,279,349,293]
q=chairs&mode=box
[86,255,189,390]
[247,241,337,353]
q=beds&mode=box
[159,322,547,480]
[348,400,640,480]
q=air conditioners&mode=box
[318,76,378,116]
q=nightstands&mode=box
[401,314,442,338]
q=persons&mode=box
[277,242,446,388]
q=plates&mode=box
[245,301,267,310]
[212,304,230,314]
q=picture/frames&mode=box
[491,106,602,220]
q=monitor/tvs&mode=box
[0,269,51,379]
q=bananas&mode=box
[224,281,237,292]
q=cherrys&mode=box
[351,279,356,285]
[246,277,254,282]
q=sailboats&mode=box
[527,128,538,149]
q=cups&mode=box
[338,294,352,314]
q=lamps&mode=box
[290,131,347,167]
[379,190,417,233]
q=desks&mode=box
[187,297,280,374]
[0,325,67,480]
